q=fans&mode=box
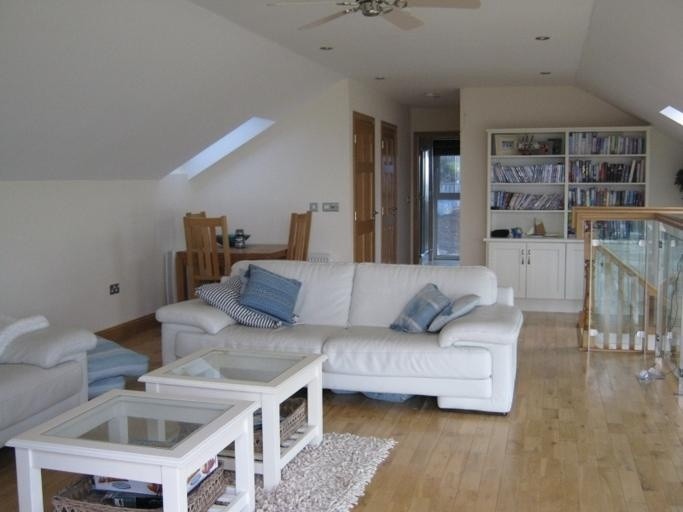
[293,0,481,31]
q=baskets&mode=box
[254,397,307,452]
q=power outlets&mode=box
[109,282,120,294]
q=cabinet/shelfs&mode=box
[483,125,652,316]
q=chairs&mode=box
[184,215,230,300]
[287,211,312,262]
[183,211,206,247]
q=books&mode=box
[491,191,563,210]
[569,214,631,240]
[569,159,644,183]
[568,187,643,208]
[491,162,565,182]
[569,133,643,154]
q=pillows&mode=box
[239,263,303,324]
[390,282,452,334]
[194,274,300,329]
[427,294,481,332]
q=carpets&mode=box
[192,433,397,512]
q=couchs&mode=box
[155,261,524,416]
[0,314,97,448]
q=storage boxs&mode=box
[223,398,308,454]
